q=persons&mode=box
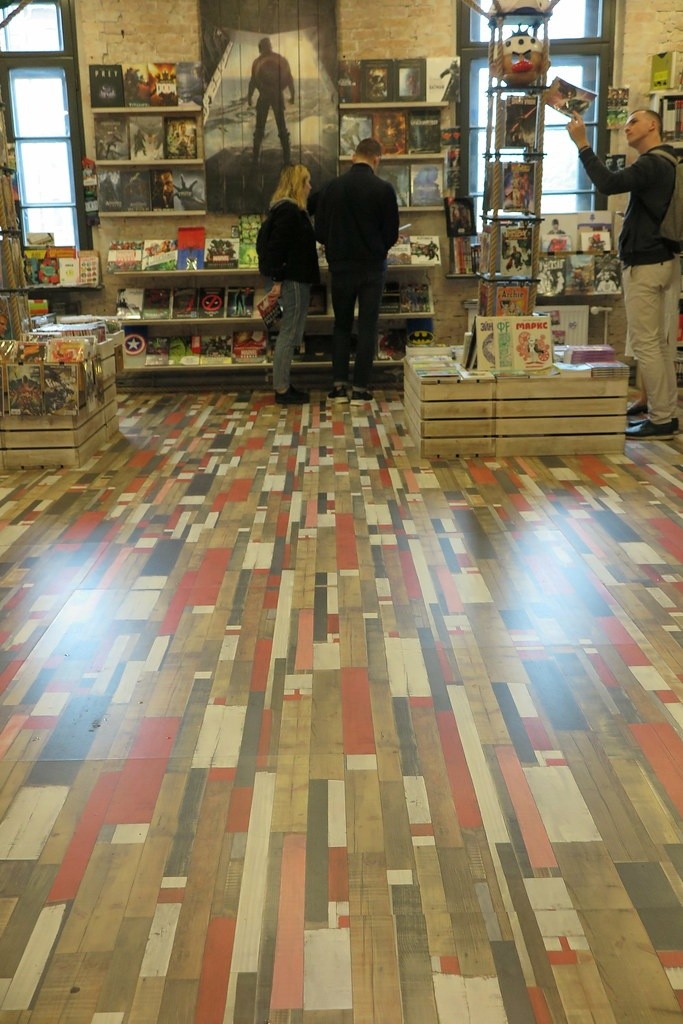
[246,38,295,166]
[315,139,400,406]
[565,110,683,440]
[175,122,192,156]
[155,170,173,208]
[256,163,321,405]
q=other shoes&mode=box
[625,399,648,415]
[275,385,310,404]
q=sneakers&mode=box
[327,387,349,403]
[624,419,675,440]
[350,391,375,405]
[628,417,679,435]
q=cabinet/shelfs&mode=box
[475,12,552,282]
[113,263,436,371]
[339,101,449,212]
[92,106,206,217]
[0,100,32,341]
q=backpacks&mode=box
[648,148,683,241]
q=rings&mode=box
[574,120,578,124]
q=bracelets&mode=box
[578,145,588,153]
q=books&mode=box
[5,321,105,416]
[106,215,441,366]
[337,55,461,206]
[89,62,207,212]
[406,77,630,380]
[649,50,683,142]
